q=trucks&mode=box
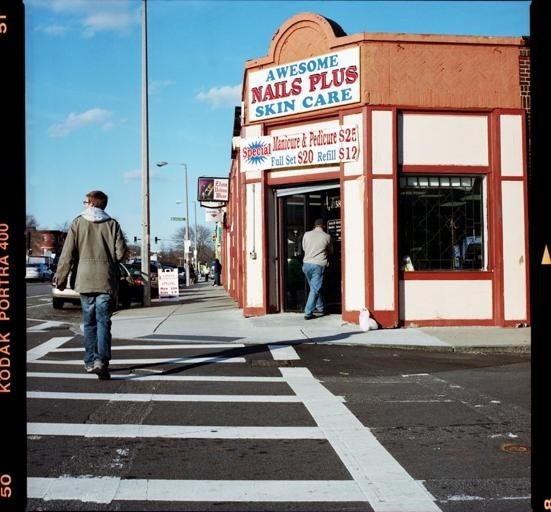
[28,256,53,265]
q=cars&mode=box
[25,263,55,283]
[49,256,136,315]
[125,258,187,298]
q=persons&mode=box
[50,190,130,382]
[300,219,335,319]
[202,259,223,287]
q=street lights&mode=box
[175,199,198,281]
[156,161,191,287]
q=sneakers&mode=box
[84,364,95,373]
[94,362,110,380]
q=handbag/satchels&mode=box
[108,261,135,292]
